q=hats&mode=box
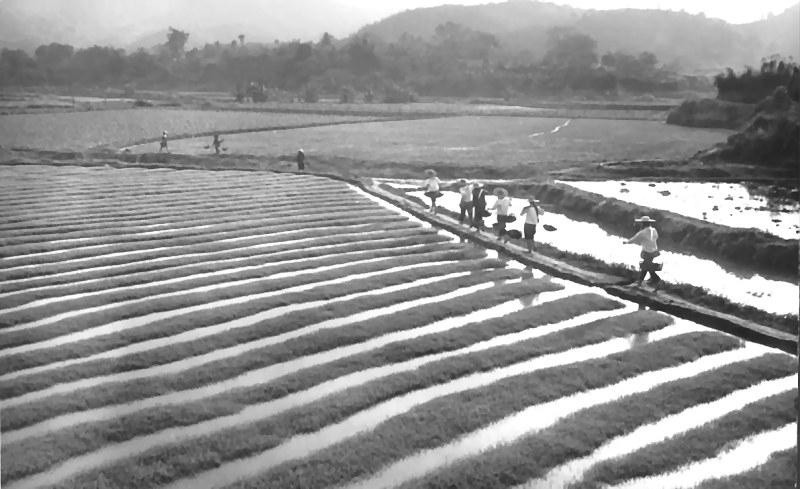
[425,169,436,177]
[493,187,508,198]
[635,216,655,222]
[457,178,483,189]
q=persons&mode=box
[486,187,512,244]
[470,182,488,233]
[158,129,171,154]
[621,215,665,294]
[211,132,221,153]
[418,169,444,212]
[296,148,306,172]
[456,177,474,228]
[520,196,544,255]
